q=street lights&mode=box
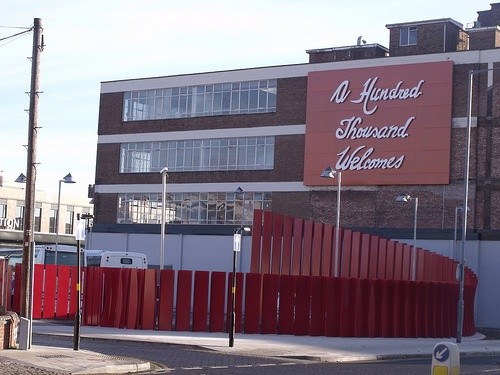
[395,192,418,282]
[456,67,499,344]
[55,173,76,265]
[320,167,343,277]
[228,227,250,347]
